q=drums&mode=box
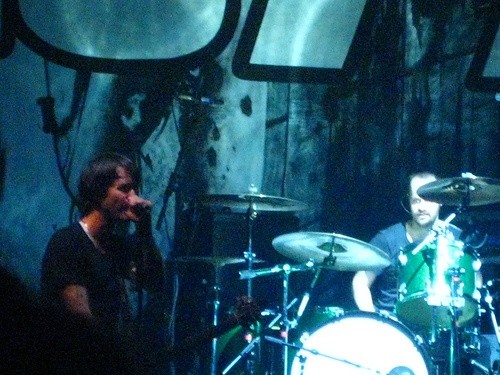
[290,311,432,375]
[218,309,284,374]
[398,239,480,331]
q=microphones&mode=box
[399,237,425,254]
[122,203,151,218]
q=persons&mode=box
[43,154,167,332]
[351,171,500,375]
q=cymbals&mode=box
[207,194,308,211]
[416,177,500,206]
[272,232,391,271]
